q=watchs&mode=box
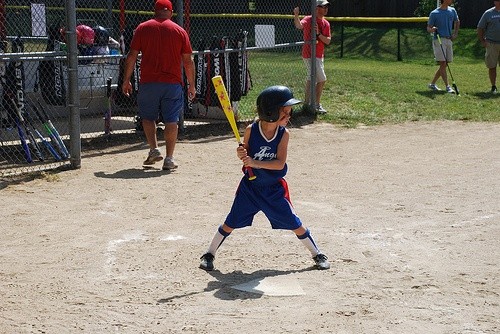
[316,32,322,37]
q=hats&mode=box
[316,0,330,7]
[155,0,172,10]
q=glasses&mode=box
[318,5,328,8]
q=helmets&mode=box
[93,26,109,44]
[257,85,302,123]
[76,25,95,44]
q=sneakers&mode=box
[429,84,442,91]
[446,86,455,93]
[200,253,214,270]
[163,160,178,169]
[312,251,330,269]
[143,148,163,165]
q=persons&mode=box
[122,0,196,169]
[427,0,460,94]
[199,84,331,270]
[477,0,500,91]
[294,0,332,114]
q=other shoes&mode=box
[314,105,326,113]
[492,88,497,95]
[303,103,313,110]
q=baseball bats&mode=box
[211,75,256,181]
[6,91,71,164]
[103,77,112,134]
